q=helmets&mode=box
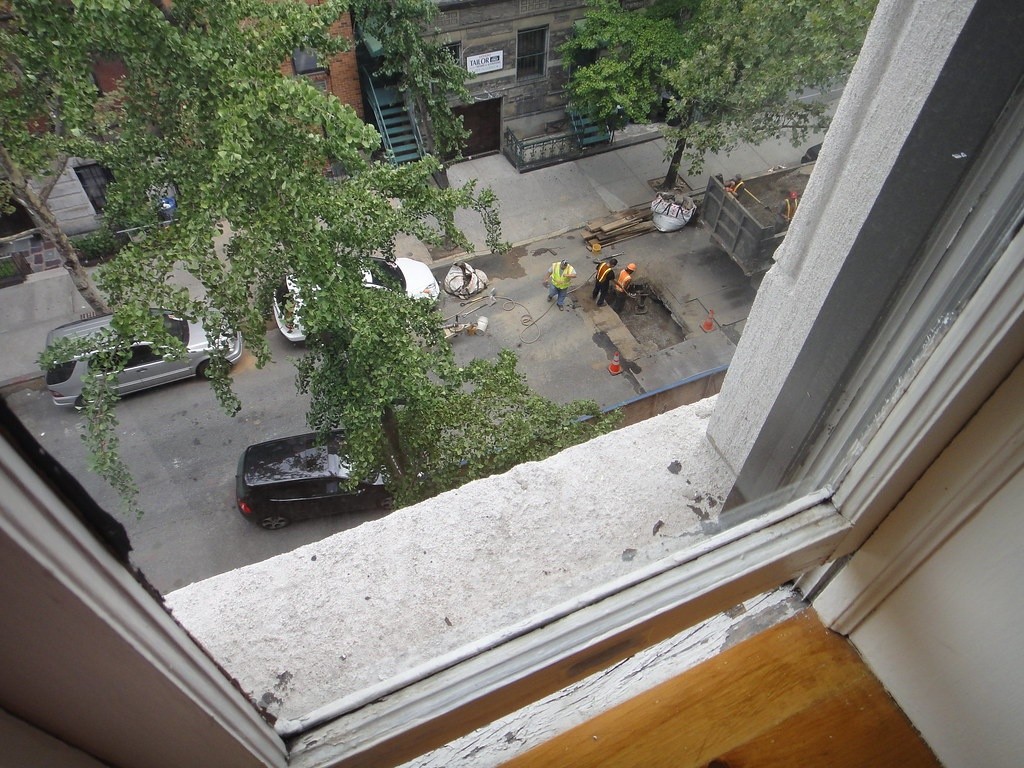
[627,263,637,272]
[789,191,798,199]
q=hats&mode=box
[735,174,742,181]
[561,259,568,266]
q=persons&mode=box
[592,259,637,313]
[543,259,577,311]
[780,191,801,230]
[723,174,744,198]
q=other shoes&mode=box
[597,302,607,307]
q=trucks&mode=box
[697,161,817,291]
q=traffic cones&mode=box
[699,310,718,334]
[607,351,624,377]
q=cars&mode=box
[273,257,440,342]
[235,428,424,530]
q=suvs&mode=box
[44,308,244,411]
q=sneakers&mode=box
[557,303,564,311]
[547,295,552,301]
